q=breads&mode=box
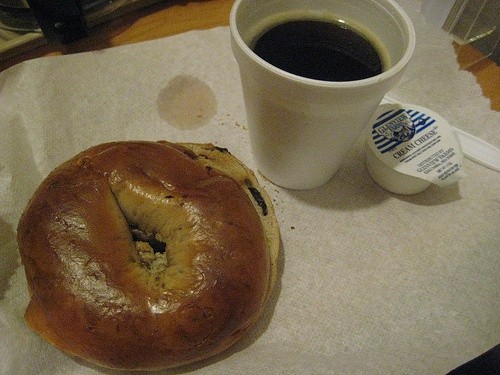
[16,139,281,372]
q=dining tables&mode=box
[0,0,500,375]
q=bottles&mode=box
[364,102,464,196]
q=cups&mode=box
[230,0,416,191]
